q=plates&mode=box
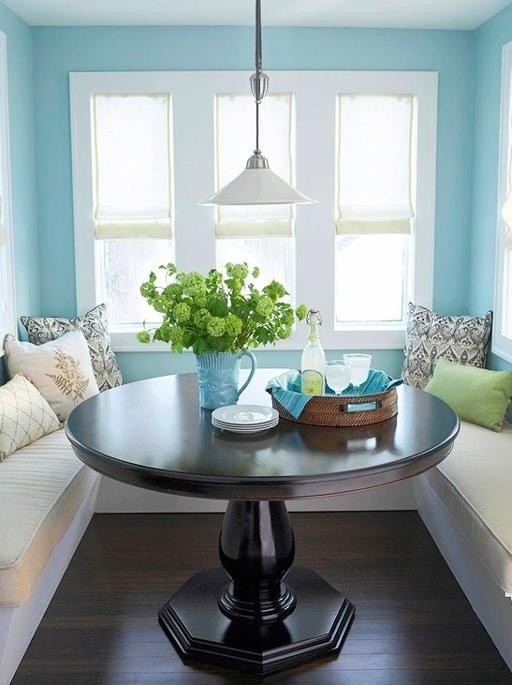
[211,404,280,433]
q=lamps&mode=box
[201,1,317,206]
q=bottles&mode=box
[299,309,327,396]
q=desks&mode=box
[61,367,459,677]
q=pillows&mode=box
[423,356,512,433]
[21,303,125,393]
[4,329,100,422]
[1,373,64,462]
[400,301,493,391]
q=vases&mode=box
[192,346,257,410]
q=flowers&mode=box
[135,262,308,356]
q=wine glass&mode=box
[344,352,372,392]
[323,359,350,395]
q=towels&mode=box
[265,366,404,420]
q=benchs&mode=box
[0,425,102,685]
[93,470,417,512]
[418,417,512,673]
